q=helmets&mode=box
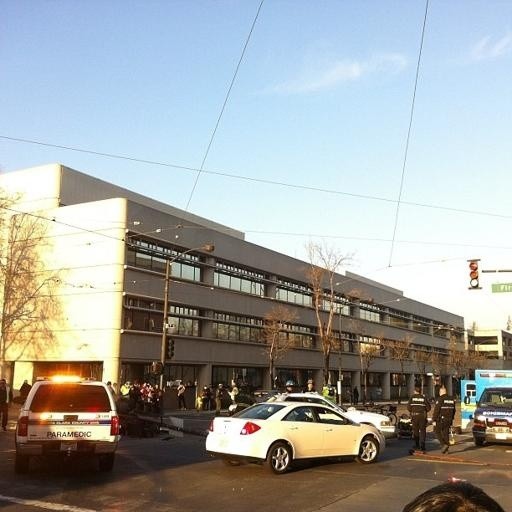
[286,380,296,387]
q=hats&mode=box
[306,379,314,384]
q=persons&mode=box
[353,385,360,406]
[302,379,316,393]
[403,479,506,512]
[431,384,457,455]
[284,380,295,393]
[104,379,243,415]
[19,379,32,406]
[0,377,13,432]
[406,386,432,451]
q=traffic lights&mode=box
[467,259,483,290]
[166,334,175,359]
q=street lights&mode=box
[160,245,215,409]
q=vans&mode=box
[461,369,512,433]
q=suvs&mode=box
[14,381,119,474]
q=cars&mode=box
[472,387,512,445]
[206,392,397,475]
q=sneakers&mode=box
[442,445,449,455]
